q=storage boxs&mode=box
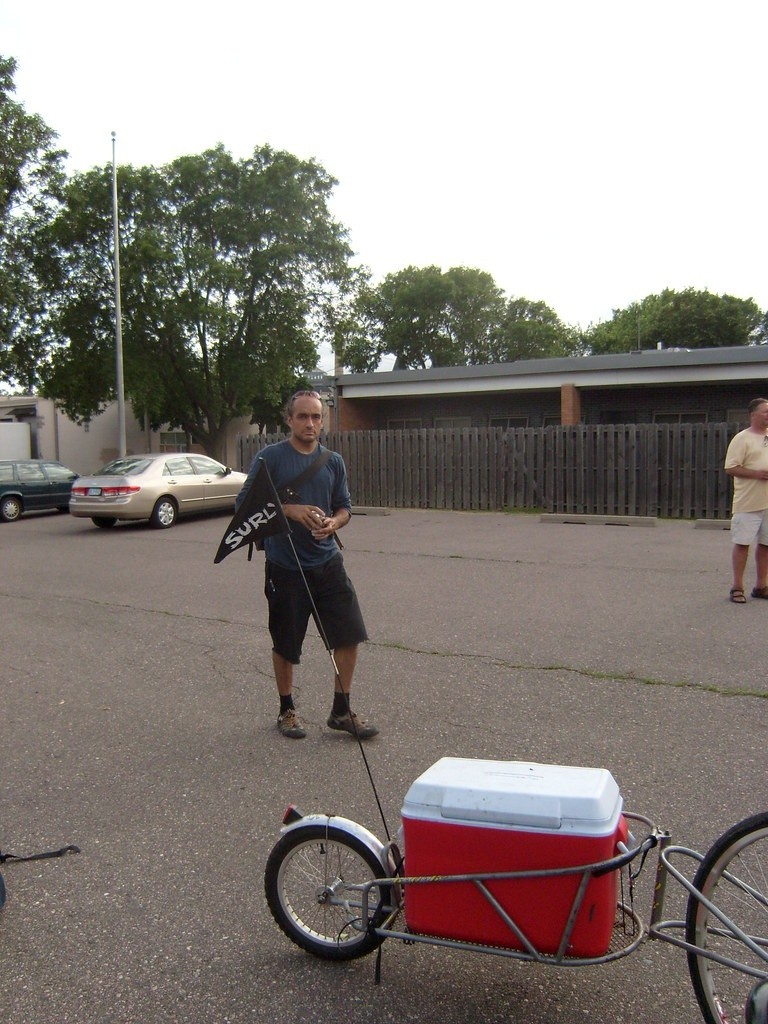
[403,754,630,957]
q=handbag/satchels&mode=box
[247,448,333,561]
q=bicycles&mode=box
[262,803,768,1024]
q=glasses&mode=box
[290,390,323,405]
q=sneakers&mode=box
[327,709,379,739]
[277,708,306,739]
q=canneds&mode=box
[311,511,325,536]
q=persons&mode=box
[236,391,378,739]
[724,398,768,604]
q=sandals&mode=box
[730,589,746,603]
[751,586,768,599]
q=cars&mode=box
[0,458,86,521]
[69,452,252,529]
